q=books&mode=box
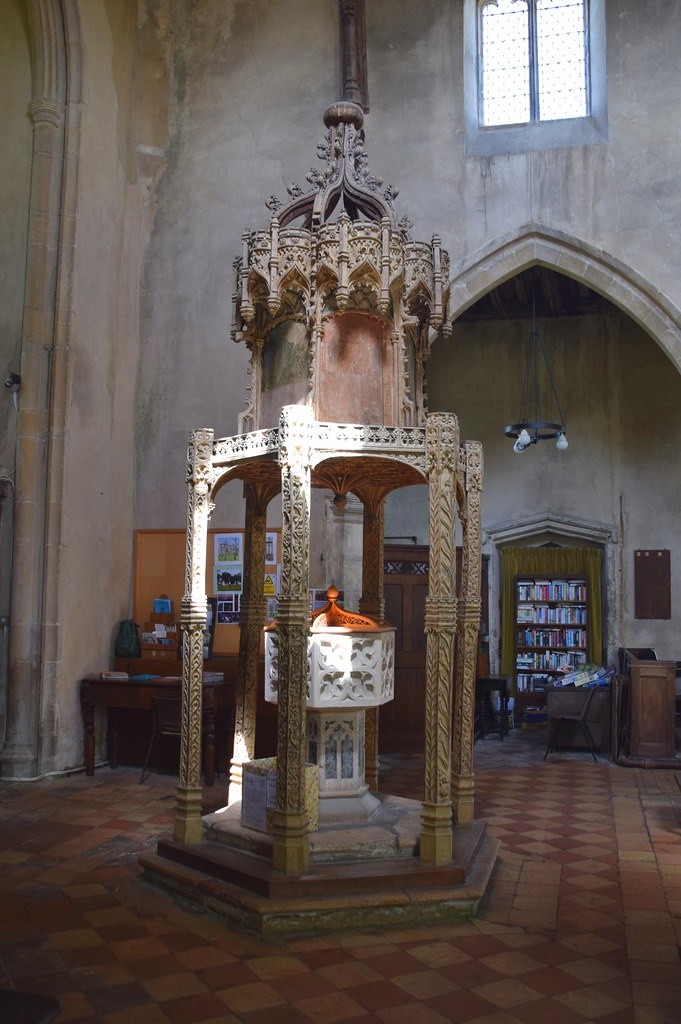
[130,671,224,682]
[154,598,171,613]
[517,673,549,693]
[518,627,588,649]
[517,605,586,625]
[553,663,614,687]
[517,578,586,602]
[516,651,588,670]
[99,671,129,679]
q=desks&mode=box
[475,672,511,741]
[79,676,238,786]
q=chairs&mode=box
[540,683,599,765]
[139,694,182,785]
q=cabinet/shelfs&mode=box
[513,574,592,725]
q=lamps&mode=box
[504,265,568,453]
